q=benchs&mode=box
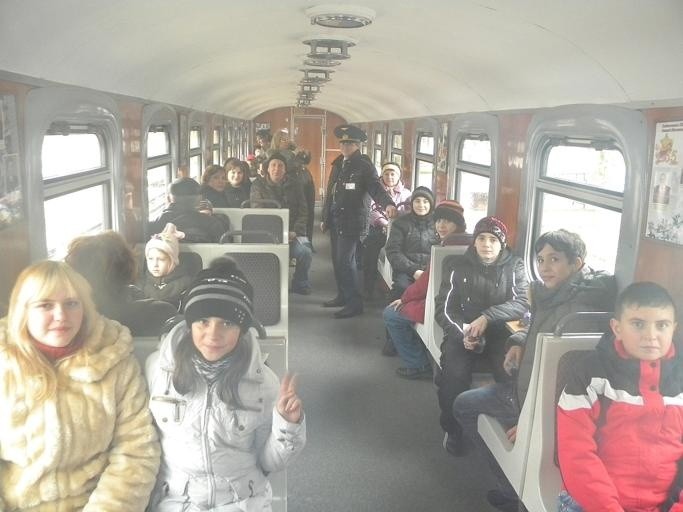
[99,175,295,511]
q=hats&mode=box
[382,161,402,174]
[166,176,203,196]
[269,153,286,164]
[410,185,434,203]
[432,199,464,224]
[473,216,507,247]
[185,269,254,334]
[333,124,368,144]
[145,233,179,266]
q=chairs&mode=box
[367,192,683,511]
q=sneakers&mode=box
[383,336,517,510]
[322,294,346,307]
[335,300,364,318]
[292,282,311,295]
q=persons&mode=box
[319,124,398,320]
[368,162,466,381]
[435,215,532,459]
[141,256,307,511]
[557,282,683,512]
[0,259,161,512]
[65,128,314,338]
[453,228,618,507]
